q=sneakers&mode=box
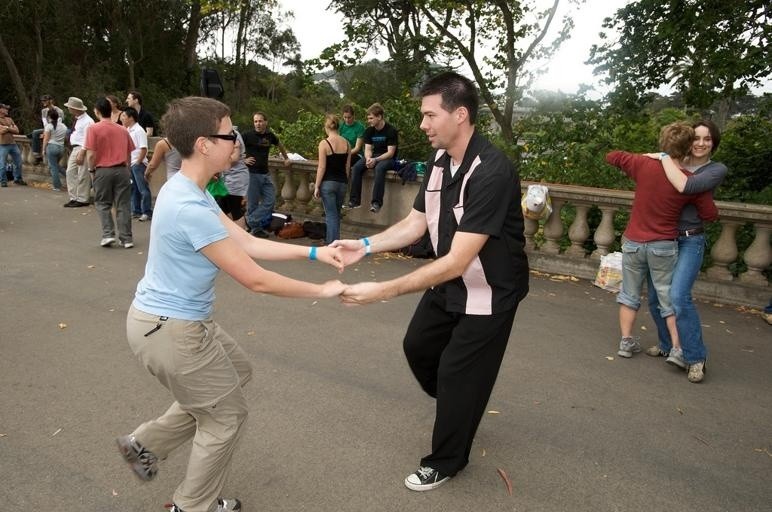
[14,179,27,185]
[131,213,140,218]
[64,200,75,208]
[646,345,669,357]
[138,214,151,221]
[101,238,115,246]
[342,202,362,210]
[68,202,88,207]
[405,466,450,492]
[52,187,62,191]
[116,433,158,481]
[164,497,242,512]
[1,182,7,186]
[119,241,134,248]
[618,336,643,358]
[665,347,688,369]
[369,202,382,212]
[255,230,268,237]
[688,356,706,383]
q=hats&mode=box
[0,104,10,109]
[63,97,88,111]
[39,95,53,101]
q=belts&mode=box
[96,162,127,167]
[677,227,703,236]
[72,145,79,147]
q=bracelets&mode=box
[309,244,317,262]
[285,156,290,160]
[6,125,10,133]
[42,150,46,153]
[659,152,667,160]
[373,156,380,166]
[362,235,373,256]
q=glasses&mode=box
[198,131,239,144]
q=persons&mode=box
[314,114,352,245]
[605,122,696,371]
[106,95,125,125]
[64,98,95,208]
[144,114,182,184]
[219,126,251,231]
[117,97,351,508]
[119,106,153,222]
[336,105,367,168]
[0,104,28,188]
[206,174,228,198]
[83,99,136,250]
[242,112,292,239]
[125,92,157,137]
[341,102,399,214]
[31,95,70,166]
[42,109,67,192]
[642,120,727,383]
[330,70,530,494]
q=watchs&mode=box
[87,168,95,177]
[88,168,96,174]
